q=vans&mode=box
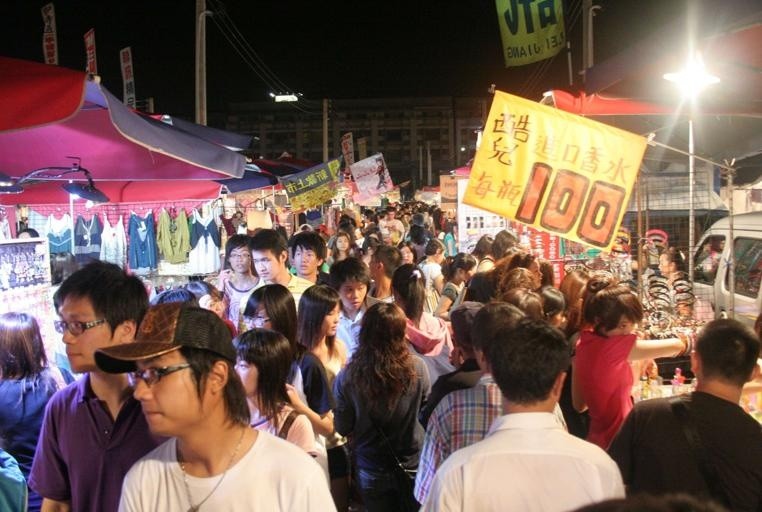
[690,213,762,344]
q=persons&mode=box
[375,157,386,190]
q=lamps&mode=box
[0,173,26,196]
[662,57,761,95]
[20,162,110,210]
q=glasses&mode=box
[128,361,196,388]
[229,252,252,261]
[53,316,111,338]
[242,313,273,328]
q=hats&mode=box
[92,302,238,376]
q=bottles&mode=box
[639,368,696,400]
[464,215,504,229]
